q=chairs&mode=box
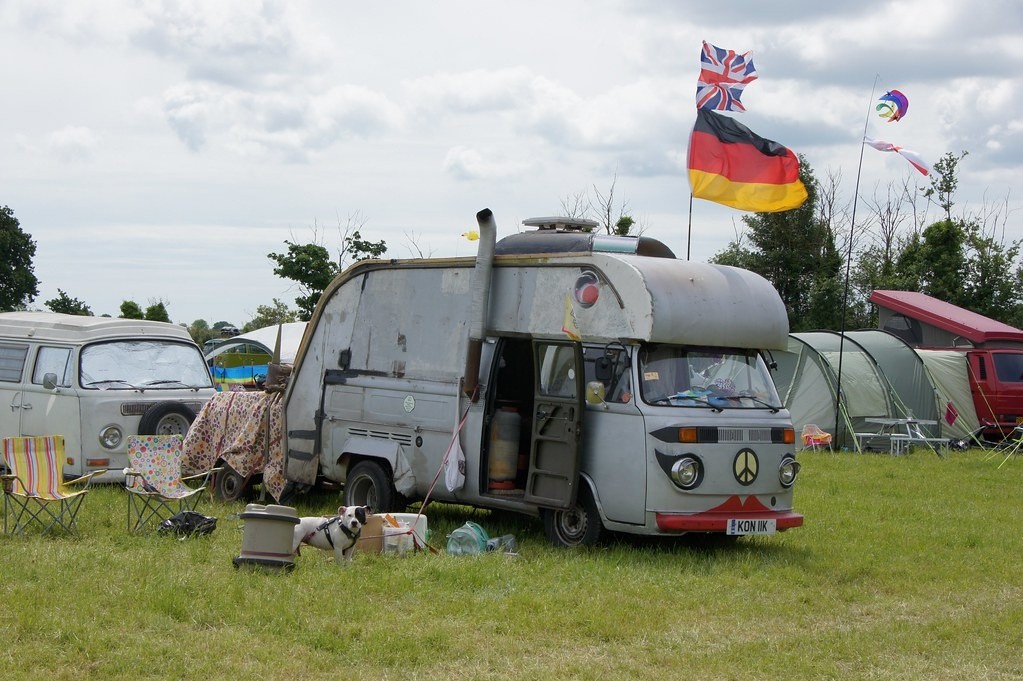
[801,425,833,453]
[123,435,224,539]
[0,435,108,540]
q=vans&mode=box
[0,310,219,486]
[286,205,808,547]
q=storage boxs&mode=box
[355,516,384,554]
[370,513,430,553]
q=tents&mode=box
[715,329,942,452]
[209,364,269,393]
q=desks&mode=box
[863,418,937,456]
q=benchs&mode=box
[889,438,951,456]
[851,433,908,457]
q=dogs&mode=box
[292,506,372,571]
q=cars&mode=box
[868,289,1023,441]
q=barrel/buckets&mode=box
[383,522,410,554]
[489,407,521,482]
[236,502,300,575]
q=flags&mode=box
[686,110,808,212]
[696,40,758,114]
[562,293,581,342]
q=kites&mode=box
[876,89,908,122]
